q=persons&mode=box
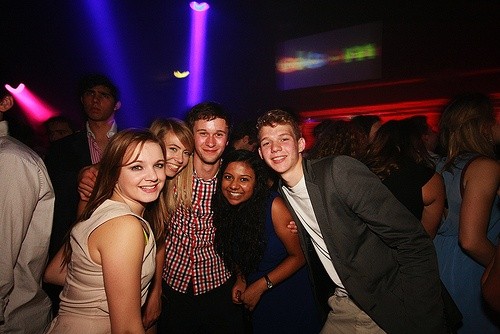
[210,150,321,334]
[78,102,298,334]
[76,117,194,333]
[44,128,166,334]
[433,92,500,334]
[256,109,438,334]
[0,75,438,334]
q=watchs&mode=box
[264,275,273,290]
